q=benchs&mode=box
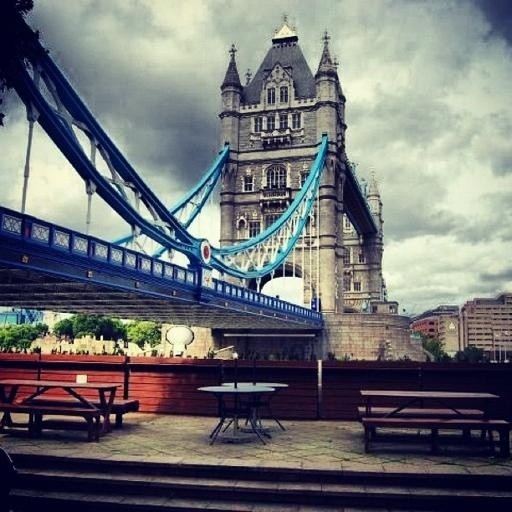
[356,404,487,438]
[363,416,512,456]
[0,403,101,443]
[21,392,139,433]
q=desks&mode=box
[220,377,290,439]
[359,387,500,449]
[0,378,120,438]
[198,386,277,446]
